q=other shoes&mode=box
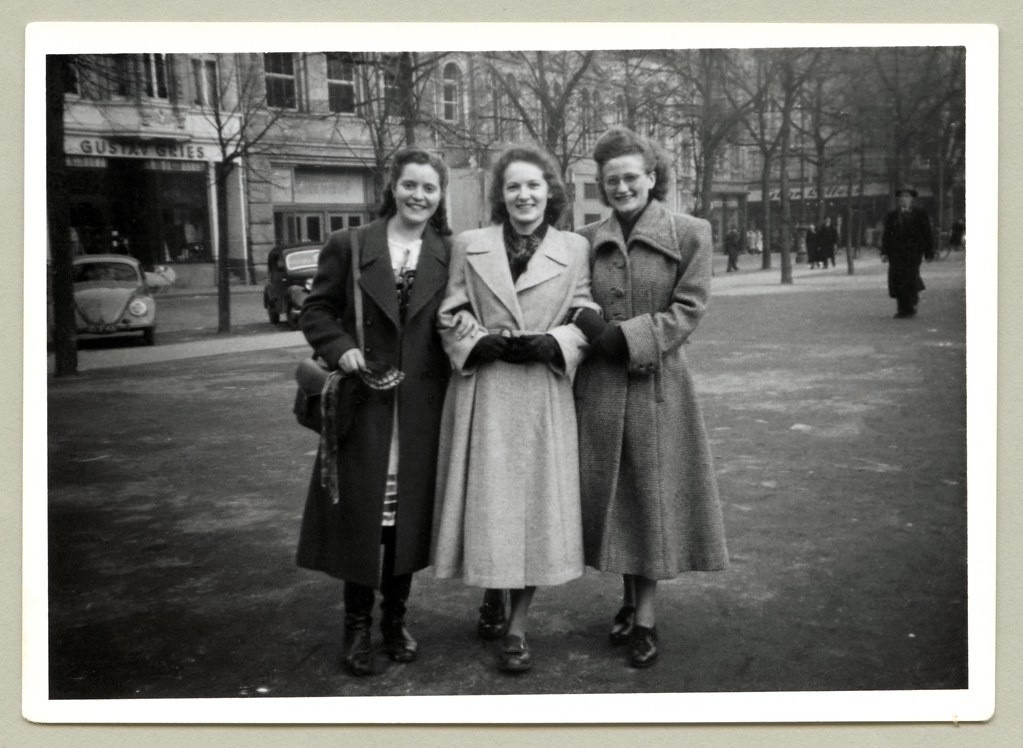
[894,307,918,317]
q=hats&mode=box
[895,184,918,198]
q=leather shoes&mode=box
[628,625,659,668]
[477,591,507,638]
[502,632,532,671]
[609,605,636,645]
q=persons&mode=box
[51,226,128,265]
[437,148,605,674]
[573,126,729,666]
[804,216,839,275]
[294,148,488,676]
[722,224,764,272]
[879,185,935,319]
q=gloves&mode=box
[502,334,545,364]
[471,335,510,362]
[577,320,630,366]
[570,307,610,343]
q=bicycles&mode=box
[924,228,952,262]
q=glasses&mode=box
[603,171,649,185]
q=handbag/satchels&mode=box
[293,357,358,444]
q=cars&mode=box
[48,252,162,345]
[261,241,325,329]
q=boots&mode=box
[343,583,386,676]
[380,577,419,663]
